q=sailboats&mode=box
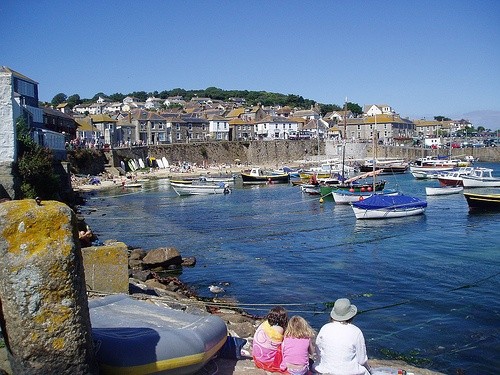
[349,114,427,219]
[249,95,408,205]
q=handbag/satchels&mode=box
[218,334,247,360]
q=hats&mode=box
[329,298,358,321]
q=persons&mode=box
[279,315,318,375]
[311,297,373,375]
[64,136,144,148]
[252,306,289,371]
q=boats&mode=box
[86,294,229,375]
[408,125,500,187]
[167,175,234,195]
[424,186,465,194]
[463,193,500,213]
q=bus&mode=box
[288,130,310,139]
